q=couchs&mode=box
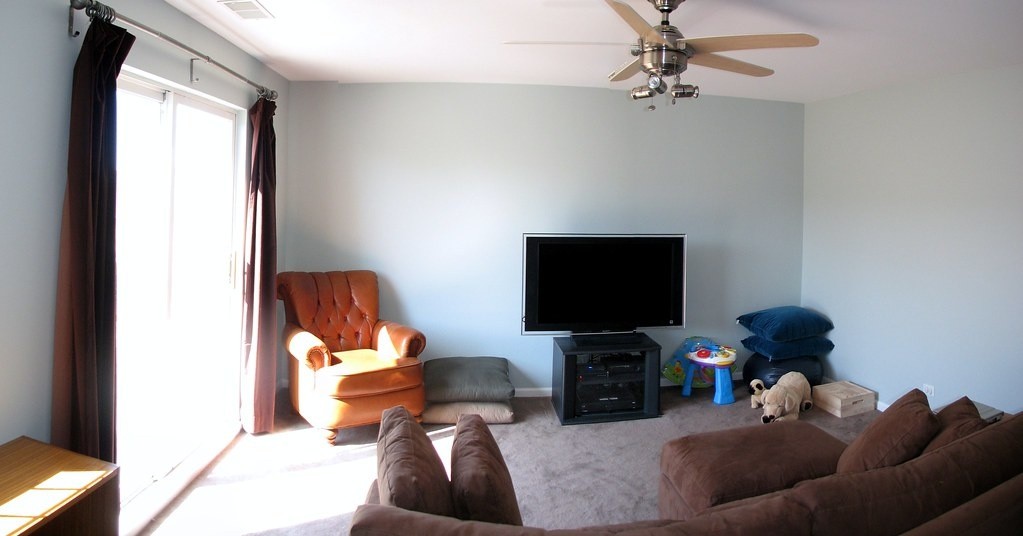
[349,474,1023,536]
[274,270,426,446]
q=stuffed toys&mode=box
[749,371,813,427]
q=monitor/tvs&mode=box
[521,232,688,335]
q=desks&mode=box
[0,436,121,536]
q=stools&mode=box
[659,419,853,521]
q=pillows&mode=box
[376,405,453,516]
[450,413,525,527]
[421,401,515,424]
[902,395,988,467]
[735,305,834,343]
[740,335,836,361]
[837,388,940,475]
[422,356,515,403]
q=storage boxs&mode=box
[812,380,875,418]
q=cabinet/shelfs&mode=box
[551,332,662,426]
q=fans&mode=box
[501,0,820,113]
